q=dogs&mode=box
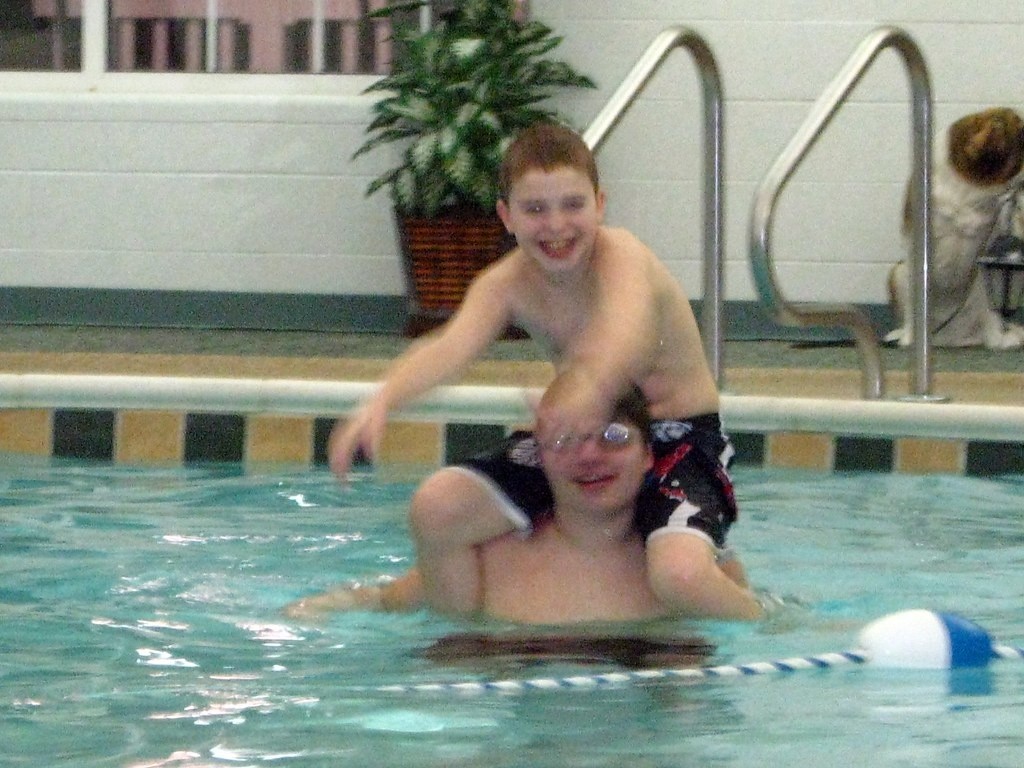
[883,105,1024,350]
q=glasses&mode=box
[542,420,631,452]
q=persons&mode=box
[328,124,759,618]
[381,365,745,620]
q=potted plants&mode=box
[346,0,601,344]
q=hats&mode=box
[533,363,652,449]
